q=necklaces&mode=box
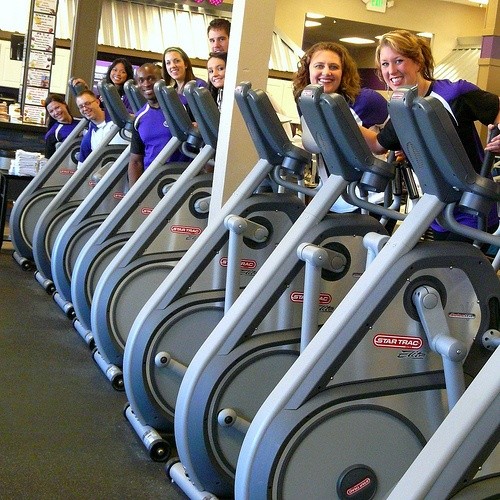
[421,78,426,93]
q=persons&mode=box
[45,19,231,192]
[292,28,500,254]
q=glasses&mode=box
[76,99,97,111]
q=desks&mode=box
[0,169,33,249]
